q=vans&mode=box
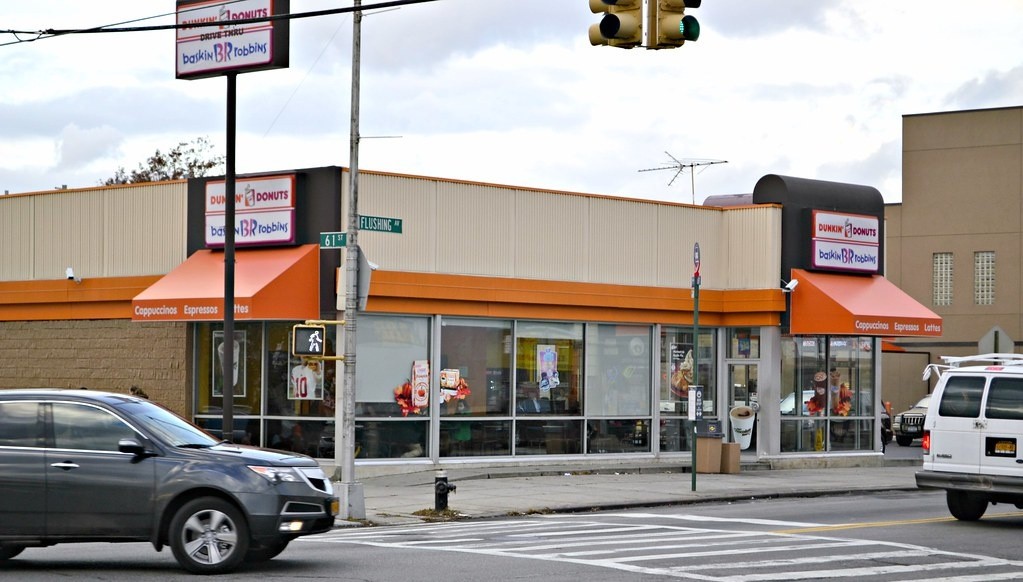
[915,351,1023,523]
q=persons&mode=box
[245,364,427,457]
[555,386,570,413]
[524,387,541,414]
[290,354,322,399]
[474,399,668,453]
[441,399,471,457]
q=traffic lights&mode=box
[599,0,643,50]
[651,1,707,51]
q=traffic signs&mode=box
[355,214,404,234]
[319,229,350,249]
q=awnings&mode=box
[132,241,323,325]
[789,267,942,338]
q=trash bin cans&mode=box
[697,433,725,475]
[721,443,741,474]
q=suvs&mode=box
[894,392,936,449]
[778,387,895,458]
[2,383,343,574]
